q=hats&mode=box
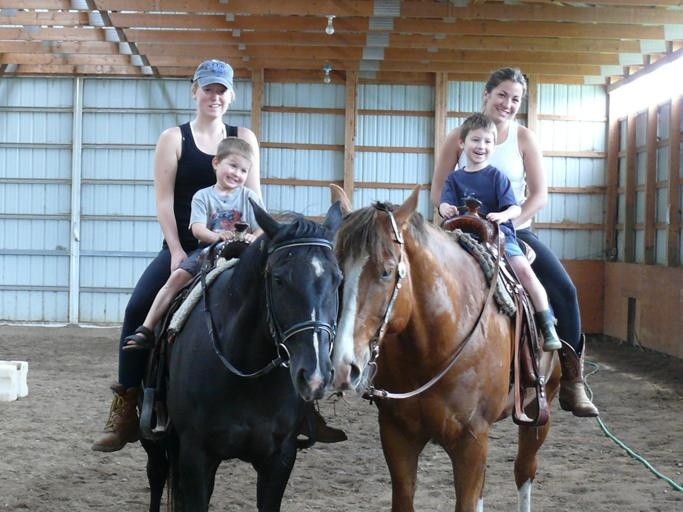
[193,60,233,90]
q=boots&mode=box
[91,384,140,452]
[300,404,348,444]
[534,311,599,418]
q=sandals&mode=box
[122,326,150,352]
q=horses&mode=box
[139,197,344,512]
[329,183,561,512]
